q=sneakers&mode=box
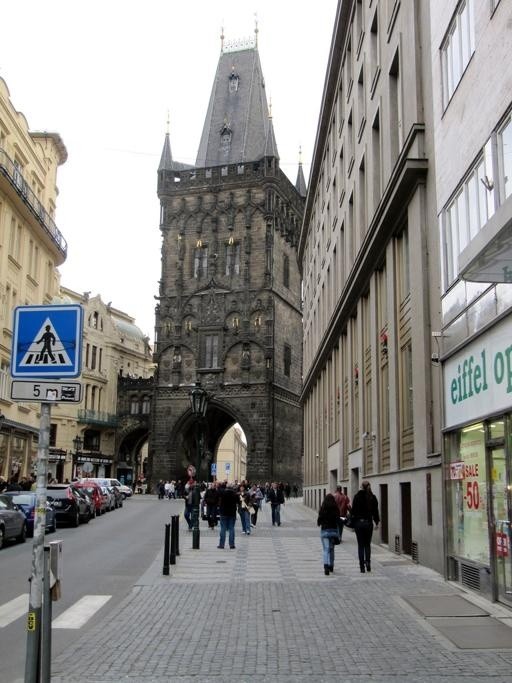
[230,546,235,548]
[241,524,256,535]
[217,546,224,548]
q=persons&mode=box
[349,480,380,573]
[317,493,341,575]
[155,477,300,549]
[0,463,70,494]
[333,485,352,543]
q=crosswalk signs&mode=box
[10,303,82,378]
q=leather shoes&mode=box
[361,568,365,572]
[325,567,329,575]
[366,562,371,571]
[330,566,333,572]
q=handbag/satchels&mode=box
[345,517,353,528]
[247,505,256,514]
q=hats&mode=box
[189,479,194,484]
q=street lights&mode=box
[187,379,207,549]
[73,435,83,477]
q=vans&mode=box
[80,478,133,500]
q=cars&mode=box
[46,482,122,527]
[0,494,28,543]
[3,490,56,533]
